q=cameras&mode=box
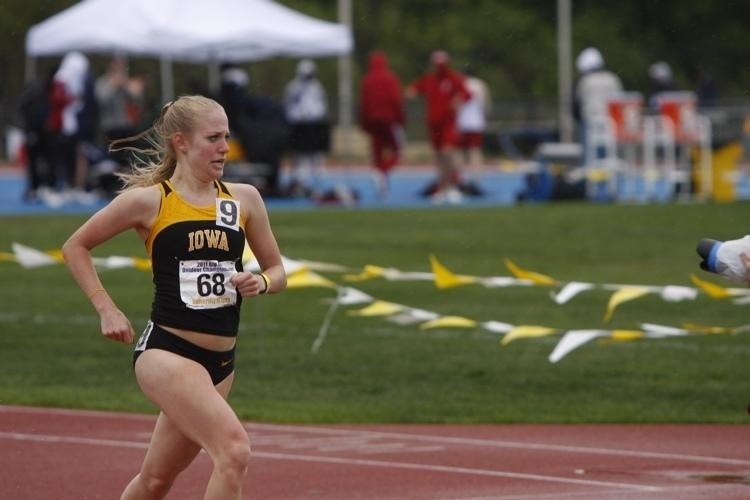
[696,235,750,280]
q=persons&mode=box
[219,46,682,201]
[18,51,145,207]
[62,96,287,500]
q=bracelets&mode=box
[255,273,271,294]
[88,287,104,298]
